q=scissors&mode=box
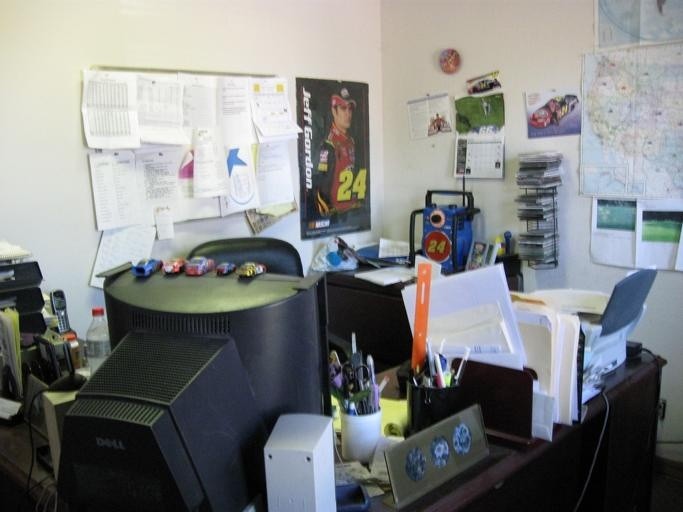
[342,361,371,385]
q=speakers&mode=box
[263,411,337,511]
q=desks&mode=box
[0,419,71,511]
[368,351,668,512]
[317,266,519,416]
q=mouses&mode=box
[49,373,86,390]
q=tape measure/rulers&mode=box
[413,263,433,371]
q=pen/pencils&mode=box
[61,342,76,376]
[411,343,472,388]
[22,358,58,385]
[329,332,391,415]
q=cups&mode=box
[340,407,383,463]
[405,379,462,437]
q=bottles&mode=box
[64,333,81,369]
[87,306,112,378]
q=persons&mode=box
[313,87,361,218]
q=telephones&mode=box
[50,289,77,340]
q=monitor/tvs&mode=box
[57,266,332,512]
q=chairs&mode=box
[185,237,356,360]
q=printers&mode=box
[565,266,659,378]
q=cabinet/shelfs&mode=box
[519,186,559,271]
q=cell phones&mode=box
[50,289,70,333]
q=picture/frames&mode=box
[295,76,372,240]
